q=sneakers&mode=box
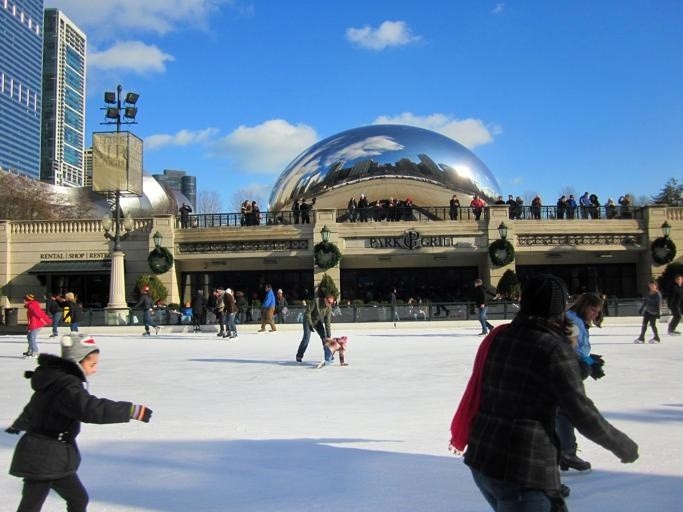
[479,331,488,335]
[490,326,493,332]
[593,322,682,344]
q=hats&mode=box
[520,274,568,317]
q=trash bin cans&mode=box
[5,307,18,327]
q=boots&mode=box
[560,444,591,472]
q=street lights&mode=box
[92,84,145,325]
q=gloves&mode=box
[5,427,20,434]
[589,355,605,380]
[128,405,152,422]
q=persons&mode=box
[22,294,53,358]
[390,287,400,321]
[2,333,153,512]
[665,275,682,334]
[531,197,541,219]
[178,202,192,229]
[496,195,523,220]
[449,194,460,220]
[557,192,632,220]
[461,272,638,510]
[319,336,348,368]
[131,285,288,338]
[408,296,427,320]
[291,198,311,224]
[348,193,414,222]
[432,285,451,317]
[294,293,336,364]
[633,280,663,343]
[472,279,495,336]
[43,292,82,338]
[240,200,261,225]
[556,290,606,469]
[471,195,483,220]
